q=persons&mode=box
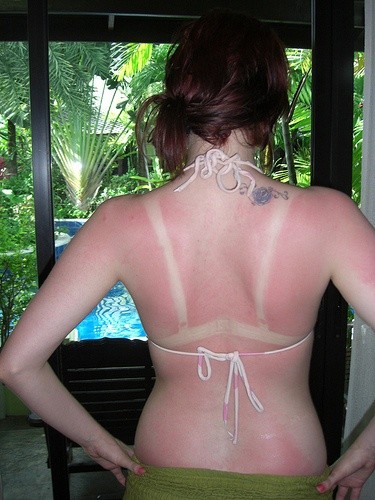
[0,5,375,500]
